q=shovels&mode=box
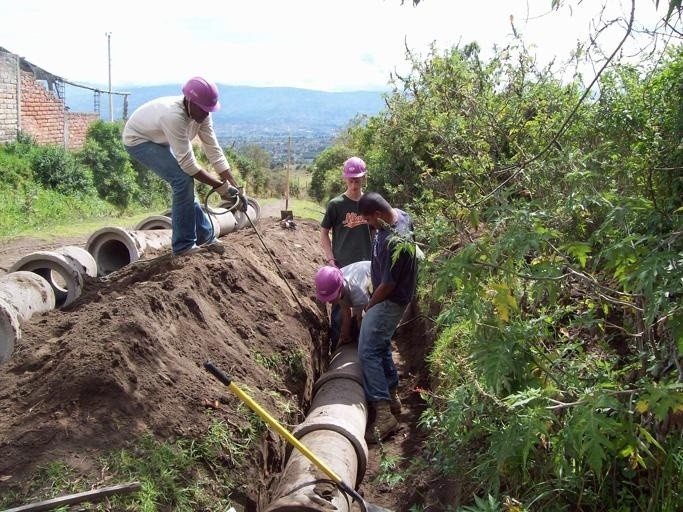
[203,362,397,512]
[281,136,293,221]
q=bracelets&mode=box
[367,304,372,308]
[327,258,336,262]
[220,176,232,181]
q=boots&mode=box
[389,388,402,413]
[365,400,400,444]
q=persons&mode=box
[318,156,377,354]
[356,190,419,443]
[313,258,373,349]
[122,76,246,256]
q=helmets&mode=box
[315,266,344,302]
[341,157,368,179]
[180,76,220,112]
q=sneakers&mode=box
[180,247,205,255]
[197,237,224,246]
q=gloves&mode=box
[232,186,247,213]
[213,179,239,201]
